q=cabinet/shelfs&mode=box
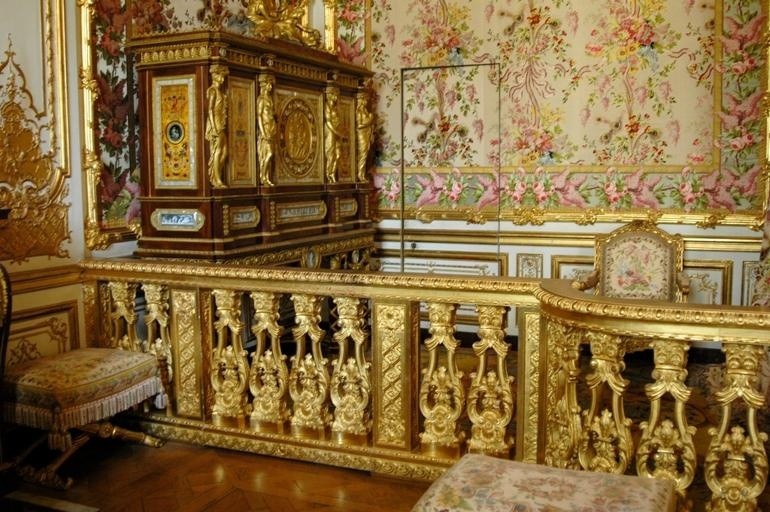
[125,25,381,352]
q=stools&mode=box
[0,347,167,490]
[410,453,674,512]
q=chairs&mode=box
[579,220,690,303]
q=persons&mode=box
[242,0,315,47]
[355,98,375,183]
[324,93,346,183]
[205,73,231,190]
[255,80,277,187]
